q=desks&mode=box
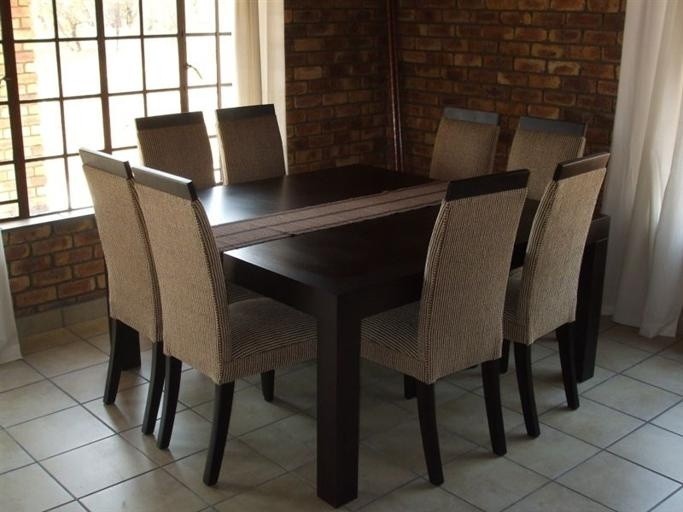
[194,164,612,508]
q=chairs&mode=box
[215,102,289,187]
[78,143,164,440]
[504,148,615,443]
[131,159,323,486]
[426,105,502,186]
[135,110,216,198]
[504,114,591,206]
[358,163,532,486]
